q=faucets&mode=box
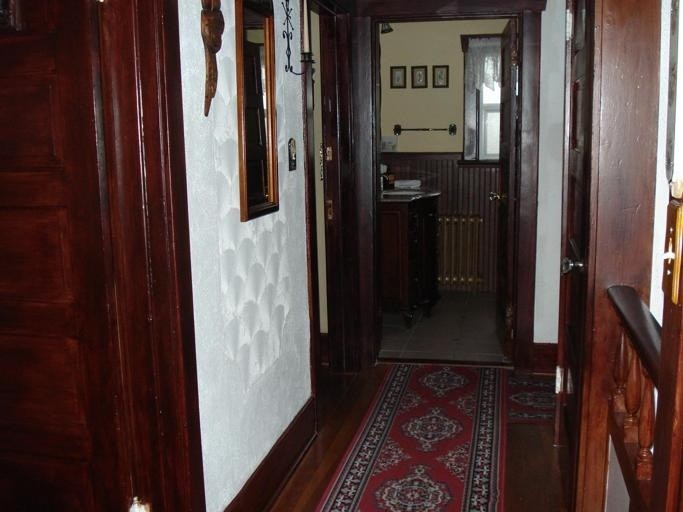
[380,174,389,182]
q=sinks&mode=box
[381,192,421,202]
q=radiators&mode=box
[436,213,486,287]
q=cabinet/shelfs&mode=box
[380,190,447,331]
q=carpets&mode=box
[506,367,555,426]
[298,361,505,512]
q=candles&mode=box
[300,0,312,55]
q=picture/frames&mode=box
[410,65,428,89]
[388,65,406,88]
[431,64,449,88]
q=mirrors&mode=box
[232,0,282,223]
